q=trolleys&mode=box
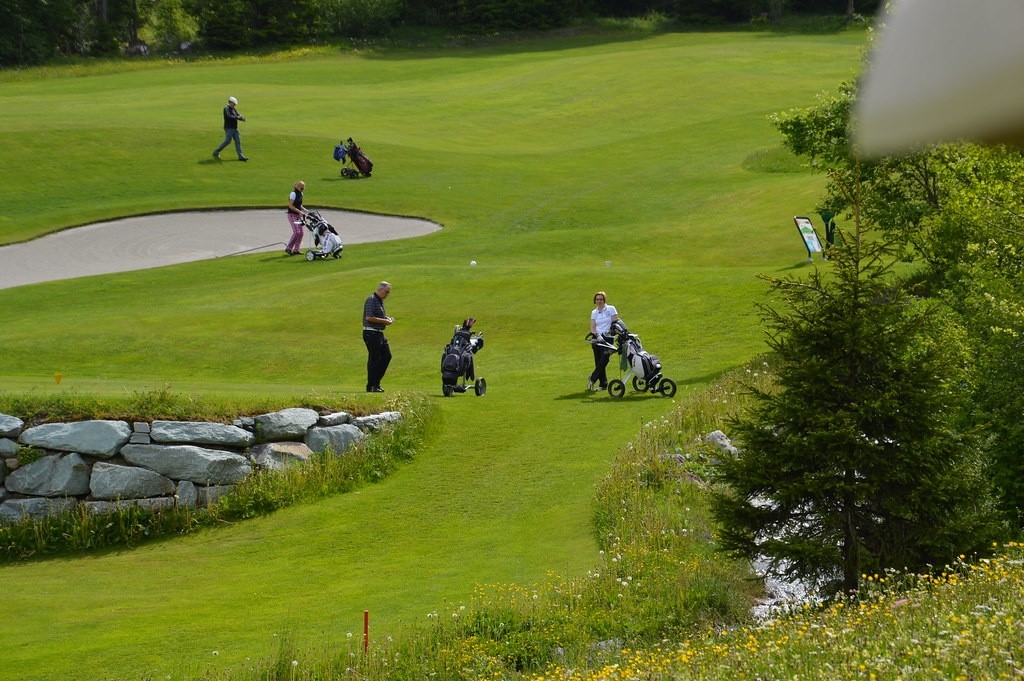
[585,318,676,398]
[333,137,374,179]
[298,210,344,261]
[441,318,487,397]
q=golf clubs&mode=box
[610,318,628,337]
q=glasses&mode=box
[384,290,390,293]
[596,298,604,301]
[298,186,304,190]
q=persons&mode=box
[585,290,618,392]
[212,97,248,161]
[361,281,394,393]
[283,181,309,257]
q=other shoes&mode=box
[213,154,222,160]
[293,252,304,255]
[285,249,293,255]
[238,157,248,161]
[366,384,384,392]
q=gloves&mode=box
[603,330,610,336]
[242,118,245,122]
[596,334,605,344]
[238,115,243,120]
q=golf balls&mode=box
[470,260,477,267]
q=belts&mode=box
[363,327,383,332]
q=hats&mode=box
[230,97,238,105]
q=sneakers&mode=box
[594,387,606,391]
[588,376,593,391]
